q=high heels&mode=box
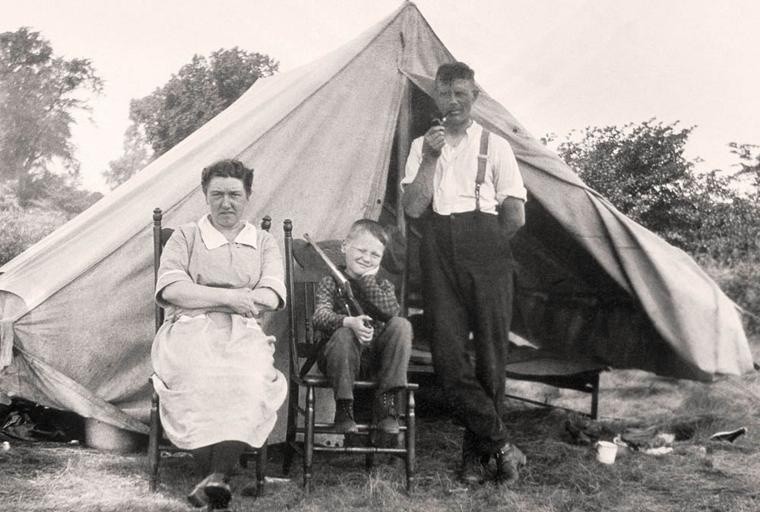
[185,472,236,512]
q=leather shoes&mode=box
[460,441,527,490]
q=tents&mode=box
[0,0,759,437]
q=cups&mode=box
[594,440,618,464]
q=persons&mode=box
[405,60,532,488]
[145,157,288,506]
[311,217,416,437]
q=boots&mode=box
[333,398,359,435]
[377,389,402,436]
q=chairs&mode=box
[153,208,272,499]
[284,218,420,500]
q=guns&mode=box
[305,234,372,348]
